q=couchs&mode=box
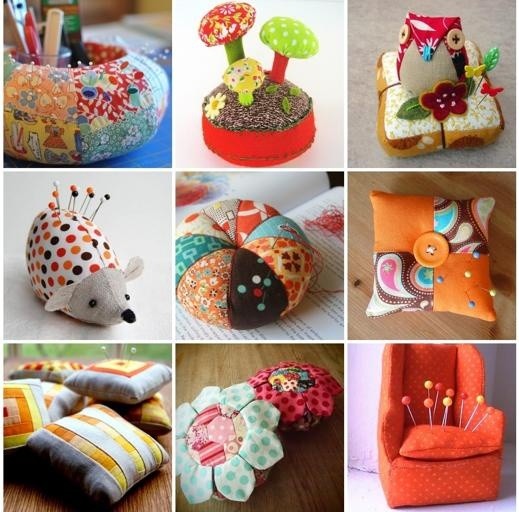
[377,344,505,509]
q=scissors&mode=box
[24,8,41,67]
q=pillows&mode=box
[4,358,172,512]
[365,189,499,322]
